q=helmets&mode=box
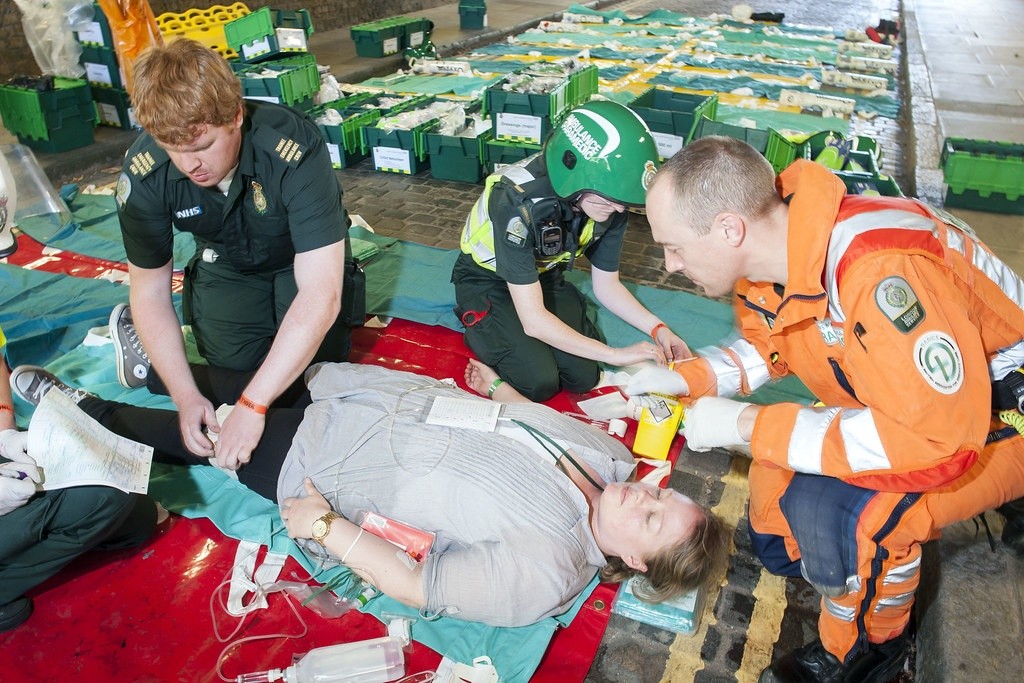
[545,99,660,203]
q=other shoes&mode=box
[0,598,33,632]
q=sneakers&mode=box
[761,634,909,683]
[109,302,151,389]
[9,365,100,408]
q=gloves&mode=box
[684,396,755,452]
[623,366,688,397]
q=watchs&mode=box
[311,510,344,548]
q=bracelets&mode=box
[651,323,669,342]
[239,396,268,414]
[0,404,15,415]
[488,379,504,398]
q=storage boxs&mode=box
[939,136,1024,217]
[0,0,905,208]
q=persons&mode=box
[9,302,745,629]
[0,326,159,641]
[450,97,693,402]
[616,135,1024,682]
[115,37,367,470]
